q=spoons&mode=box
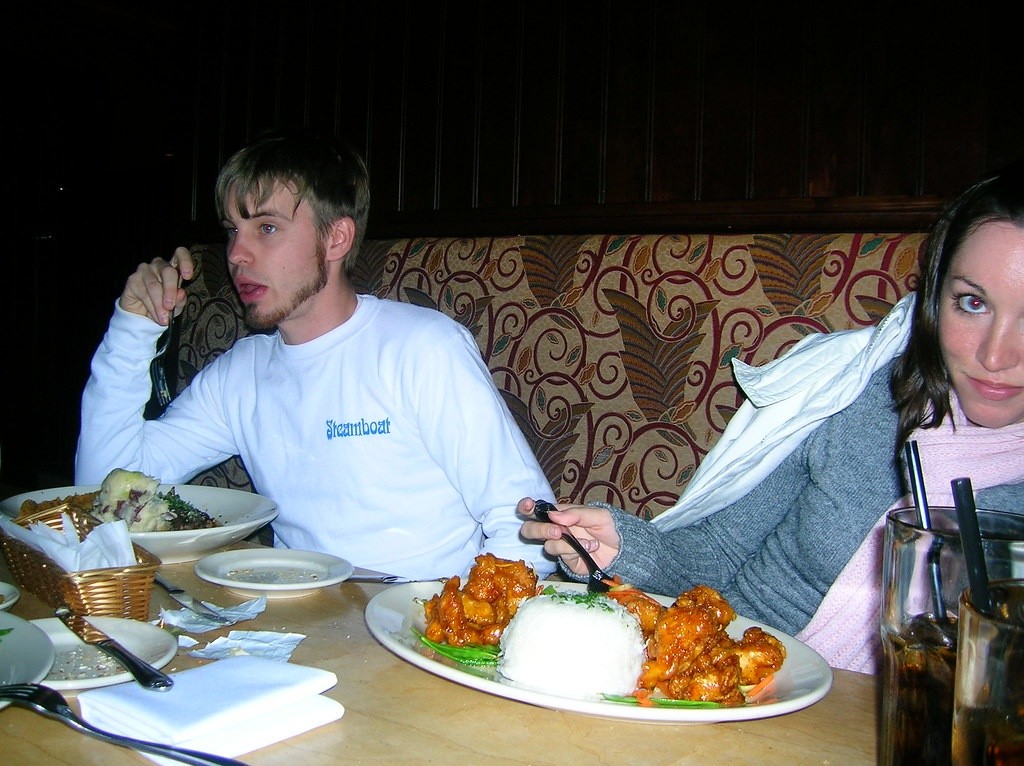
[533,500,609,594]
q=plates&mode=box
[0,580,19,611]
[0,611,54,710]
[28,615,177,690]
[365,580,832,726]
[195,548,354,599]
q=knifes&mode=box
[154,574,230,623]
[345,575,447,583]
[56,605,172,691]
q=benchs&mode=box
[179,194,959,522]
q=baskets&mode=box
[0,500,163,644]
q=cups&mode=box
[878,506,1024,766]
[952,578,1024,766]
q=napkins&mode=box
[78,654,345,766]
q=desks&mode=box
[0,542,881,766]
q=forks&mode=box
[0,684,248,766]
[148,266,181,407]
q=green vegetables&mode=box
[409,583,725,708]
[153,486,215,531]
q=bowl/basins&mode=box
[0,484,281,565]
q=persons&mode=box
[515,171,1024,675]
[73,135,561,590]
